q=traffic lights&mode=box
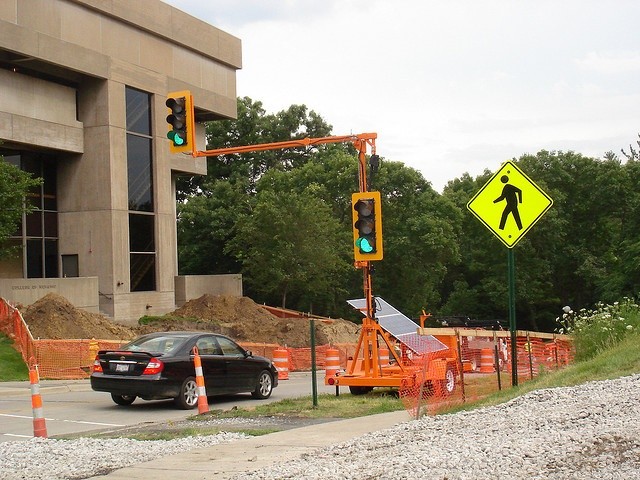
[353,198,377,254]
[165,96,188,146]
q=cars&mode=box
[90,330,279,410]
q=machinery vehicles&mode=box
[324,315,473,397]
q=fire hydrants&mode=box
[89,337,100,374]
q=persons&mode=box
[198,341,210,355]
